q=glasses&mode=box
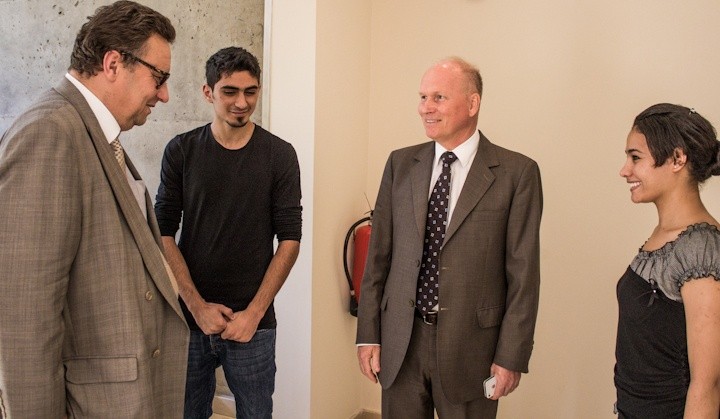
[118,49,170,89]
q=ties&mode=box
[109,137,126,178]
[415,151,458,317]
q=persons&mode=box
[154,48,303,419]
[355,58,543,419]
[0,0,190,419]
[614,103,720,419]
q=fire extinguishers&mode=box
[349,209,375,317]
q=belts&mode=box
[415,310,438,326]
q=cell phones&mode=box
[482,376,498,400]
